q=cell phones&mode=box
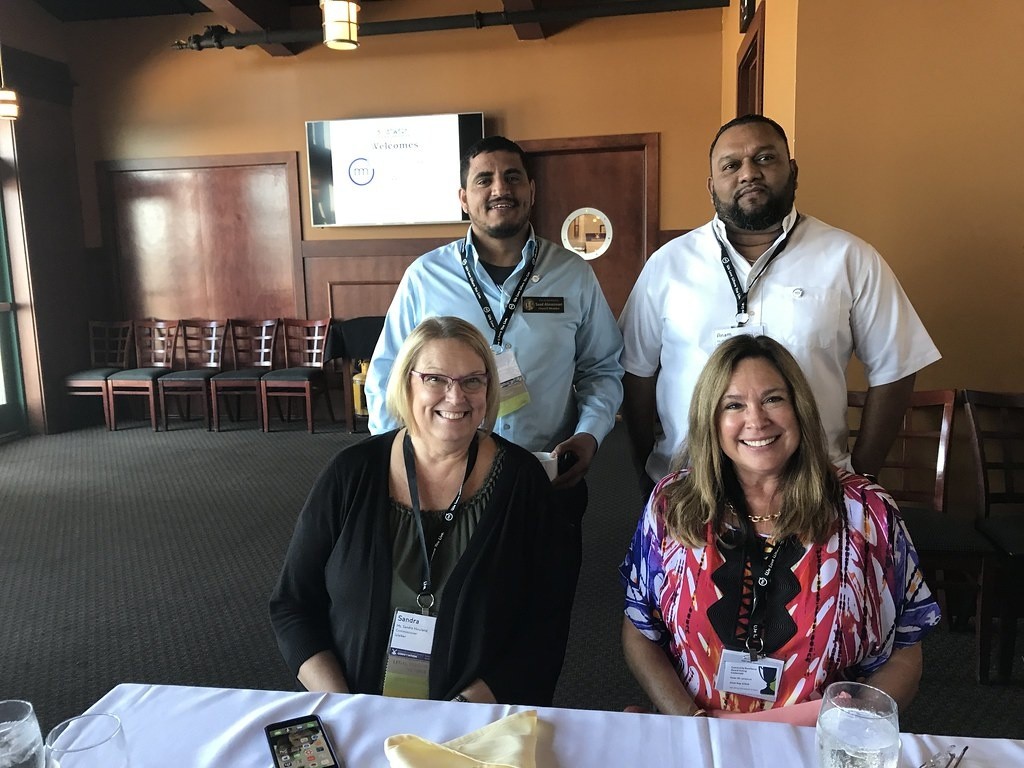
[264,714,341,768]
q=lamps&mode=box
[319,0,362,50]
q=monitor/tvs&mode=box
[305,111,485,228]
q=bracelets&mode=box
[454,694,470,703]
[859,473,878,483]
[692,709,707,718]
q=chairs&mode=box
[56,319,132,430]
[107,320,178,431]
[260,315,338,435]
[846,388,994,686]
[962,390,1024,686]
[157,319,229,432]
[210,319,279,433]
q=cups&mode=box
[44,713,130,768]
[0,699,46,768]
[530,452,558,482]
[815,682,900,768]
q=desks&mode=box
[43,683,1024,768]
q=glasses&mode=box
[917,745,969,768]
[409,370,491,394]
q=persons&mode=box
[364,137,625,707]
[619,337,943,728]
[618,112,940,513]
[268,318,582,708]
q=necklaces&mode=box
[745,257,758,263]
[725,499,781,523]
